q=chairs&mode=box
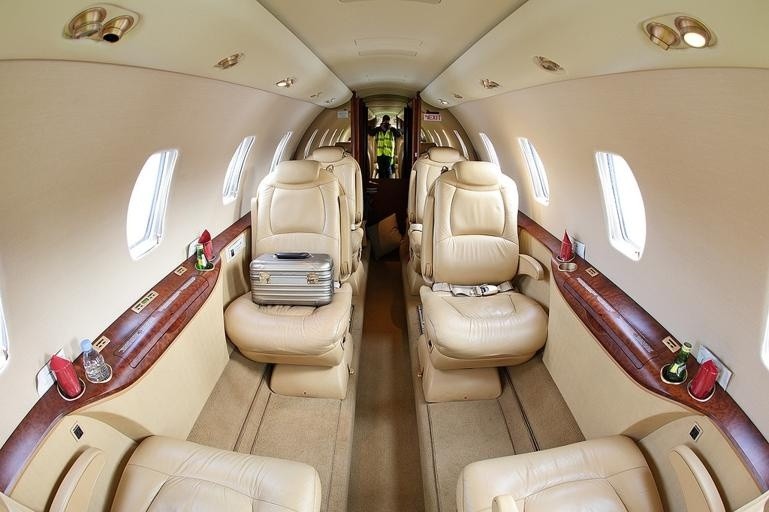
[307,148,364,294]
[407,148,468,297]
[455,435,769,512]
[224,161,353,399]
[421,162,548,403]
[0,437,321,512]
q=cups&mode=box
[560,240,576,261]
[690,357,721,399]
[51,358,81,398]
[202,241,214,261]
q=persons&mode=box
[367,114,401,179]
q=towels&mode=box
[199,230,214,261]
[49,355,82,399]
[691,360,719,398]
[559,230,573,261]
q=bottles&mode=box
[196,243,212,269]
[664,342,692,382]
[80,339,110,382]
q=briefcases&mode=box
[249,252,335,307]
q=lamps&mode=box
[68,5,136,44]
[218,54,241,68]
[645,17,710,50]
[539,56,559,74]
[482,79,500,89]
